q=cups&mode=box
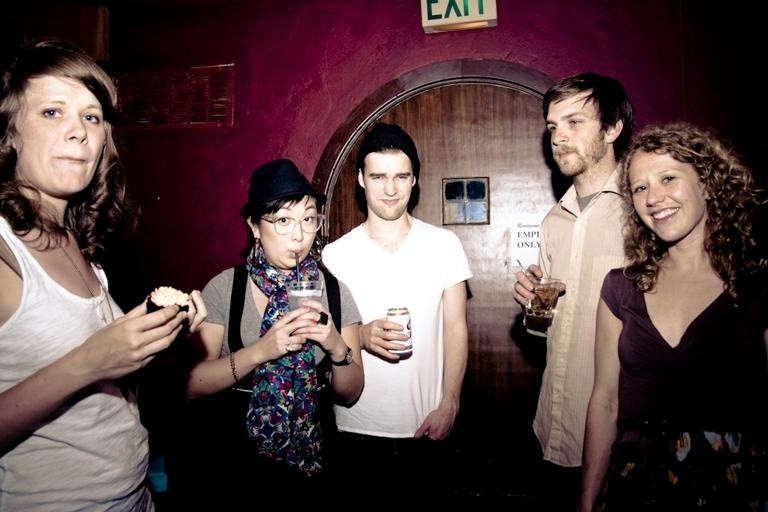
[287,280,324,330]
[523,276,560,332]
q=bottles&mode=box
[387,307,413,355]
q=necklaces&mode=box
[43,223,115,326]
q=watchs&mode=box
[329,347,353,367]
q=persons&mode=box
[579,123,768,512]
[186,156,363,512]
[0,43,208,512]
[320,124,474,512]
[511,70,635,512]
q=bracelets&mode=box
[229,350,241,384]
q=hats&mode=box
[238,157,327,218]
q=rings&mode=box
[318,311,329,326]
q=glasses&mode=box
[265,214,327,238]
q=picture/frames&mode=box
[443,177,489,226]
